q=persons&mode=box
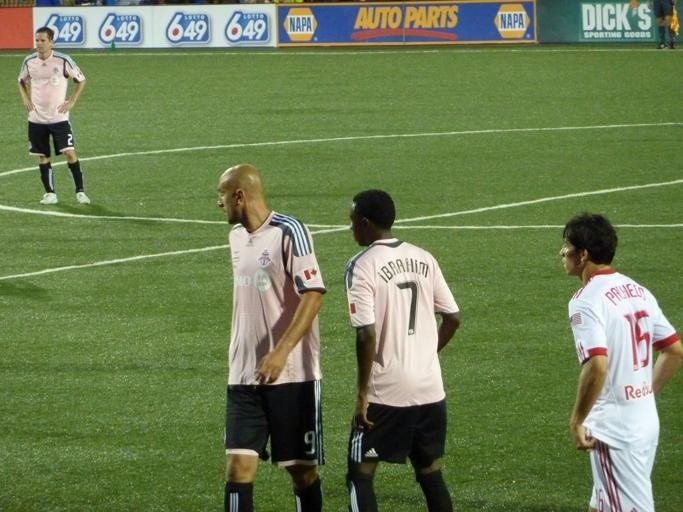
[555,208,683,512]
[214,160,329,512]
[16,27,91,205]
[342,188,463,512]
[651,0,678,49]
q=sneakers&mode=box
[77,190,90,204]
[656,42,675,49]
[39,192,58,205]
